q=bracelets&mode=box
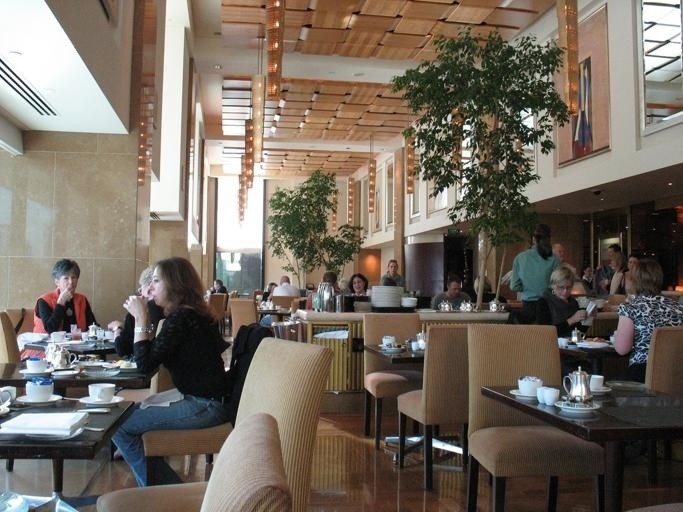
[134,328,154,334]
[112,327,123,330]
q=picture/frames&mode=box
[556,3,612,168]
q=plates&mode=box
[577,342,609,349]
[15,359,137,408]
[26,428,84,443]
[510,387,612,413]
[378,344,387,348]
[371,286,418,308]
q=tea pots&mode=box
[562,366,593,403]
[571,327,585,344]
[44,342,61,363]
[53,348,77,370]
[88,321,101,338]
[438,298,504,313]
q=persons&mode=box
[272,276,300,311]
[430,275,471,311]
[110,257,234,488]
[210,280,228,312]
[348,273,368,297]
[108,266,166,390]
[553,243,639,297]
[260,283,278,319]
[380,259,404,288]
[508,224,561,322]
[471,276,507,304]
[606,259,683,384]
[305,272,341,311]
[19,259,101,363]
[534,263,597,384]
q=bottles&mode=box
[266,300,270,308]
[417,333,425,349]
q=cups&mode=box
[518,375,604,406]
[129,295,148,309]
[558,338,568,347]
[71,325,112,341]
[382,336,395,345]
[412,342,419,351]
[0,386,16,402]
[610,336,615,344]
[51,331,65,342]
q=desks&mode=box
[2,401,137,500]
[481,385,680,512]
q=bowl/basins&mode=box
[385,348,402,355]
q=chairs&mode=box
[396,322,500,490]
[2,291,308,486]
[466,323,604,510]
[292,286,683,449]
[200,413,292,510]
[96,337,334,510]
